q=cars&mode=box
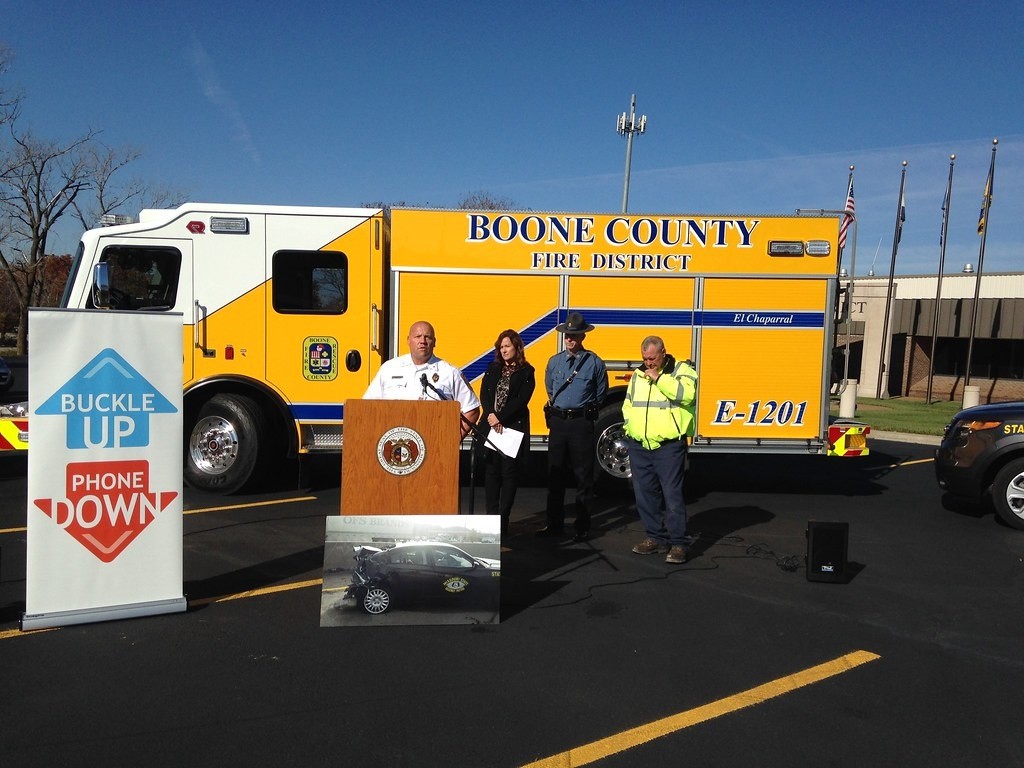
[934,401,1024,530]
[344,541,500,616]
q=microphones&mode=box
[422,373,427,393]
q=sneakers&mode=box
[665,545,689,563]
[632,539,668,554]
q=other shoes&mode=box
[574,529,588,541]
[535,526,564,536]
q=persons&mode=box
[536,313,608,542]
[622,336,698,564]
[478,330,536,544]
[361,321,481,444]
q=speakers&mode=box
[805,519,849,583]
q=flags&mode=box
[838,174,855,250]
[898,178,905,244]
[977,152,995,236]
[940,171,953,246]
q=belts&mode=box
[551,407,586,418]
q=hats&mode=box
[557,312,594,334]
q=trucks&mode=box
[0,202,872,495]
